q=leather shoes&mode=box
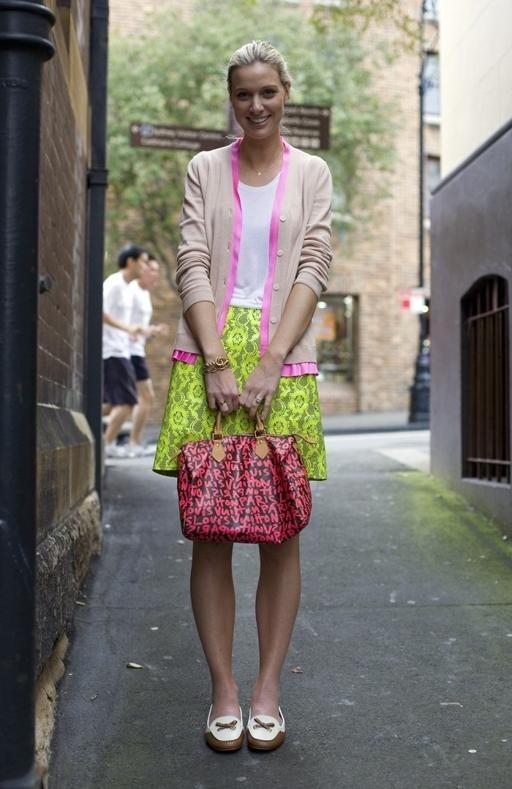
[245,705,286,751]
[204,701,245,752]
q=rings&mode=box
[219,399,226,407]
[255,395,262,405]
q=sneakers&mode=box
[103,438,158,460]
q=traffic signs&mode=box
[229,101,330,150]
[130,122,229,151]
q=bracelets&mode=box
[201,355,232,375]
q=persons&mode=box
[103,242,149,460]
[125,255,170,456]
[150,37,335,755]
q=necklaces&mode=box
[245,146,278,177]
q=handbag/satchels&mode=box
[178,408,312,546]
